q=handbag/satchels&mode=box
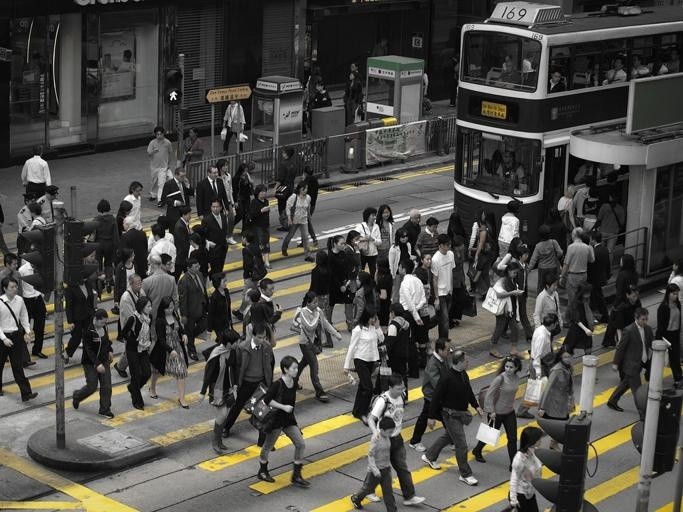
[279,193,297,229]
[523,379,542,408]
[249,405,275,435]
[359,224,368,250]
[475,419,500,447]
[482,286,507,316]
[478,374,504,410]
[244,382,273,424]
[301,326,322,354]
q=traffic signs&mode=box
[203,84,253,105]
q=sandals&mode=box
[266,264,272,270]
[510,350,517,354]
[63,352,69,364]
[489,351,503,358]
[178,398,189,408]
[148,387,157,399]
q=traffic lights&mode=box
[18,223,56,296]
[530,413,592,512]
[65,217,101,289]
[163,66,183,106]
[631,383,683,474]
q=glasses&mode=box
[506,355,517,362]
[401,235,407,238]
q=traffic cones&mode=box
[341,146,359,174]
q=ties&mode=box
[212,180,217,194]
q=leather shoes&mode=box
[23,361,36,368]
[36,352,48,359]
[22,393,38,402]
[607,401,623,412]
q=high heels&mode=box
[471,447,486,463]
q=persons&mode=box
[117,50,134,72]
[245,303,277,348]
[177,257,209,361]
[409,337,452,451]
[413,252,438,355]
[383,302,412,406]
[274,146,298,232]
[354,207,382,274]
[308,251,334,349]
[538,343,575,420]
[644,283,683,389]
[222,322,276,452]
[146,126,173,207]
[413,216,440,257]
[113,247,139,343]
[187,232,209,286]
[561,227,596,329]
[297,37,461,139]
[18,243,49,360]
[0,203,9,255]
[420,349,482,486]
[447,212,473,293]
[402,208,422,255]
[587,230,611,323]
[294,165,319,247]
[115,200,134,239]
[172,206,192,285]
[497,236,523,270]
[242,287,273,341]
[148,216,175,252]
[28,202,46,231]
[16,191,37,270]
[113,272,147,378]
[187,224,212,272]
[149,253,173,276]
[199,329,241,454]
[573,175,596,226]
[468,51,673,93]
[201,198,228,280]
[222,100,246,154]
[72,308,115,418]
[351,416,398,512]
[92,199,121,293]
[561,281,595,355]
[0,276,39,402]
[376,203,396,264]
[148,295,190,409]
[281,181,316,262]
[63,276,95,364]
[216,159,237,245]
[496,150,528,192]
[183,127,204,167]
[431,233,457,339]
[327,234,352,333]
[533,274,560,352]
[21,143,51,201]
[232,229,267,321]
[343,304,385,427]
[161,167,194,234]
[115,215,149,281]
[343,230,362,278]
[294,290,342,402]
[528,225,564,296]
[615,284,643,382]
[388,226,417,303]
[606,306,655,412]
[366,374,426,506]
[37,184,59,224]
[247,184,272,270]
[256,355,313,488]
[471,354,523,473]
[597,190,625,254]
[516,313,559,419]
[226,172,255,246]
[196,165,231,221]
[119,295,153,411]
[375,255,393,325]
[398,259,426,378]
[78,249,100,348]
[0,252,37,368]
[141,254,180,355]
[582,186,600,233]
[123,181,144,220]
[468,208,497,302]
[507,425,545,512]
[468,207,483,294]
[350,270,378,328]
[509,246,534,344]
[557,185,578,244]
[146,223,177,280]
[489,262,523,358]
[601,253,639,348]
[232,163,249,208]
[497,200,521,258]
[206,272,233,342]
[668,257,683,345]
[258,278,283,325]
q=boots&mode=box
[213,422,222,453]
[220,437,226,449]
[292,463,310,488]
[257,461,275,482]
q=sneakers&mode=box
[351,496,361,509]
[422,455,441,470]
[366,493,381,502]
[281,249,288,257]
[99,411,114,418]
[408,442,427,452]
[73,390,79,409]
[113,362,127,377]
[403,495,426,506]
[304,257,315,262]
[549,440,562,452]
[459,474,479,486]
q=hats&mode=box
[45,185,59,194]
[22,192,39,199]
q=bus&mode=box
[454,0,682,268]
